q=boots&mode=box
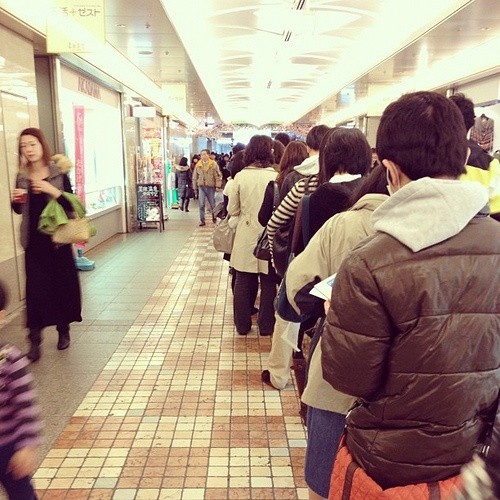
[56,325,71,349]
[27,333,41,359]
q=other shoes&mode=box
[179,207,183,211]
[250,307,258,315]
[199,219,205,227]
[239,329,249,336]
[212,218,216,223]
[185,210,189,212]
[261,369,279,390]
[260,331,272,336]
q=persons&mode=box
[0,279,46,500]
[9,129,87,361]
[174,90,500,500]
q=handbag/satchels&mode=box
[186,187,194,198]
[292,316,325,425]
[50,215,90,244]
[252,181,289,261]
[275,274,300,323]
[273,174,316,277]
[328,434,495,500]
[213,213,233,253]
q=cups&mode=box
[16,189,28,204]
